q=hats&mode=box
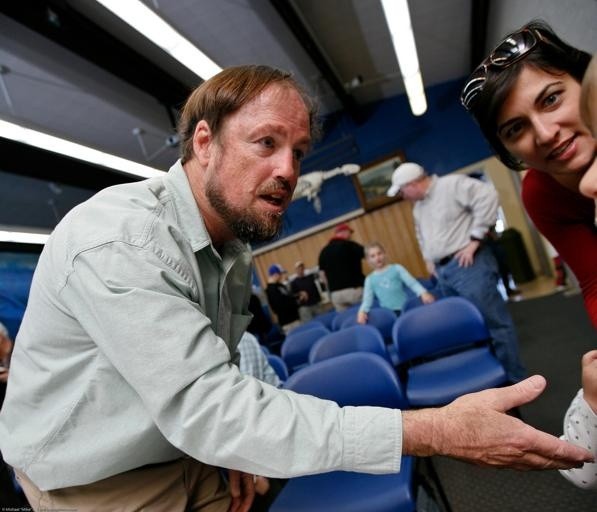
[385,162,424,199]
[268,264,288,278]
[335,224,354,236]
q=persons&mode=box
[265,264,310,327]
[487,224,525,298]
[0,62,597,512]
[357,243,436,325]
[289,260,319,321]
[459,17,597,337]
[246,294,265,347]
[386,161,530,387]
[318,224,364,311]
[553,49,597,495]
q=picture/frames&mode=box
[350,152,409,214]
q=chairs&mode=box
[260,277,456,378]
[266,351,438,510]
[266,354,289,384]
[392,297,507,510]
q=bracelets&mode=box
[470,237,483,243]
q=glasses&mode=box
[460,29,540,113]
